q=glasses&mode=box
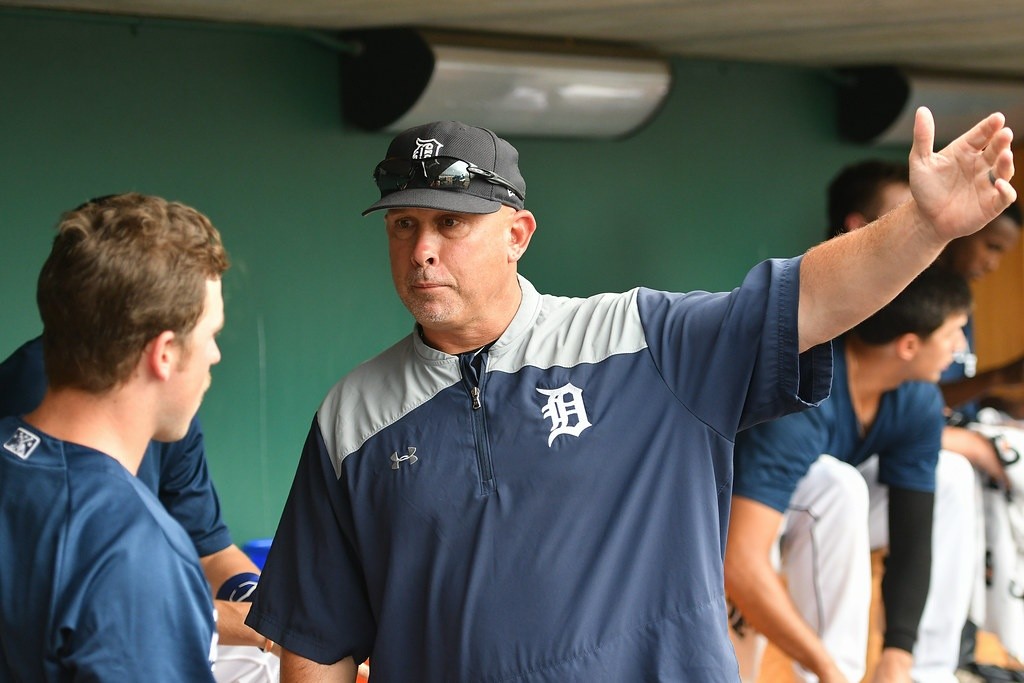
[373,156,525,202]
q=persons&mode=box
[243,104,1024,683]
[0,189,281,683]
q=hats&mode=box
[361,120,528,214]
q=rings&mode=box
[986,169,997,186]
